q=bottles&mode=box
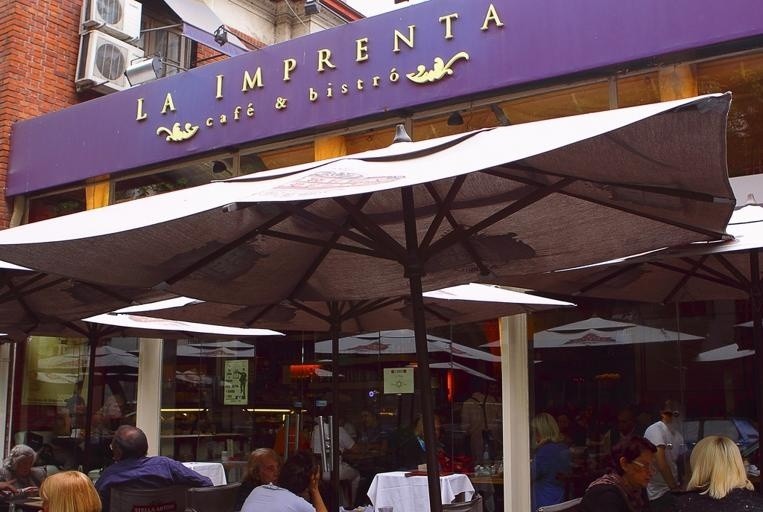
[481,444,490,464]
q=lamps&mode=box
[448,111,464,125]
[124,52,189,86]
[304,0,350,24]
[212,160,233,176]
[213,24,260,50]
[393,124,412,142]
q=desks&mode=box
[366,471,475,512]
[181,462,228,487]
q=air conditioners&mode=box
[78,0,142,48]
[75,30,144,95]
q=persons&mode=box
[1,397,760,512]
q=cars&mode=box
[681,418,759,456]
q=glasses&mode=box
[631,461,656,471]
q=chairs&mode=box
[537,497,582,512]
[555,466,611,503]
[442,493,483,512]
[108,484,190,512]
[184,480,242,512]
